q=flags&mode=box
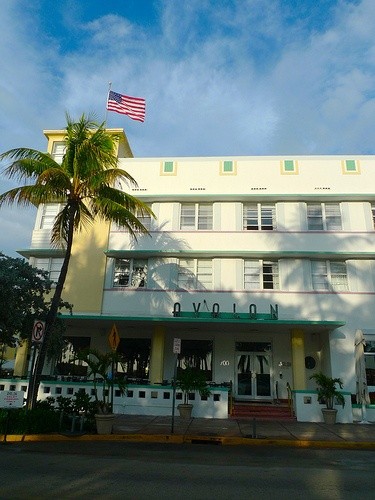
[106,90,146,123]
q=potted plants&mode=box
[309,373,345,424]
[170,362,211,417]
[78,349,129,433]
